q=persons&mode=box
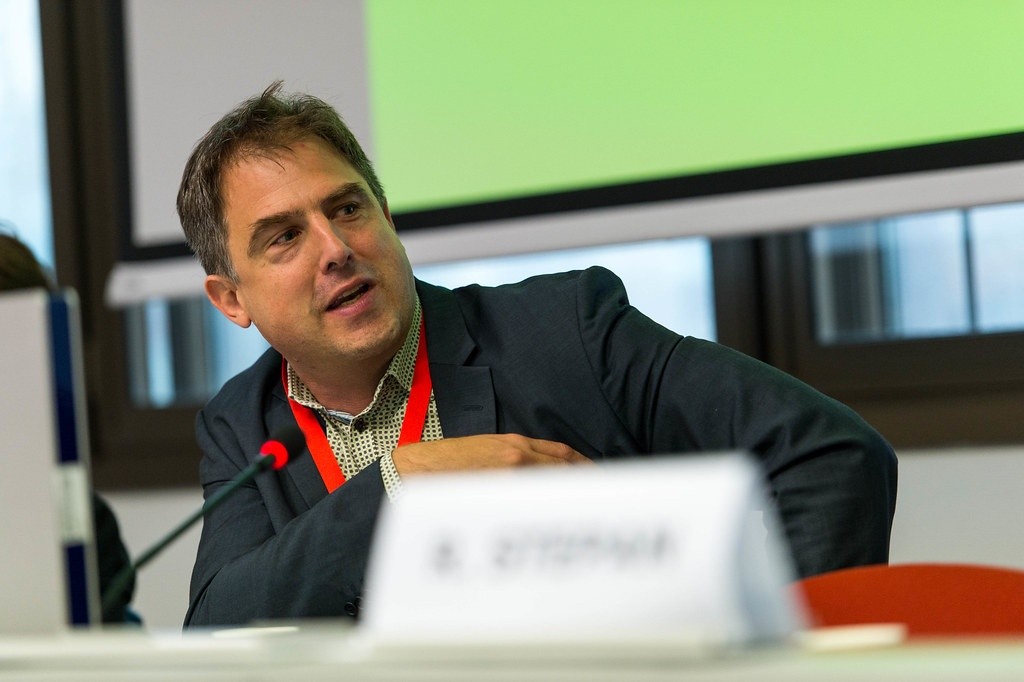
[0,232,142,627]
[176,94,897,631]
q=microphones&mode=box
[101,427,307,621]
